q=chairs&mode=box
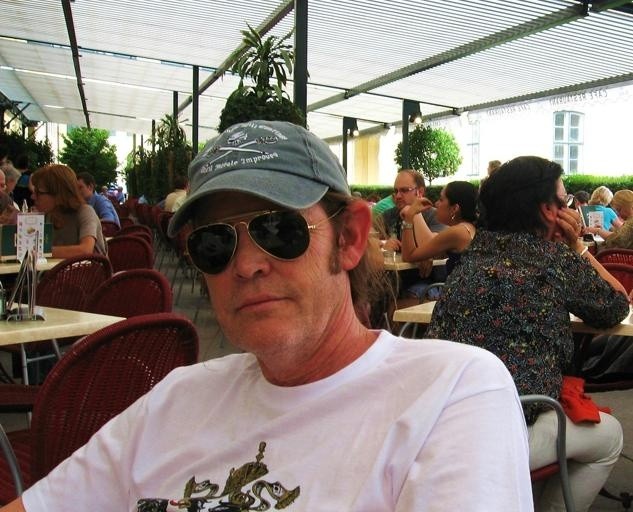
[101,195,154,272]
[1,314,198,507]
[594,248,633,305]
[35,252,114,312]
[84,268,173,320]
[520,394,576,512]
[154,205,209,324]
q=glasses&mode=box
[394,186,417,193]
[183,205,345,274]
[560,194,575,206]
[35,186,51,199]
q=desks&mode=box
[392,299,633,337]
[0,251,92,278]
[0,303,127,433]
[378,239,450,271]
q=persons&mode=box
[0,154,188,273]
[349,156,632,331]
[425,156,629,511]
[0,120,537,512]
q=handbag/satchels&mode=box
[559,375,612,424]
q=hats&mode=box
[167,120,352,239]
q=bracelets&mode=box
[576,244,589,259]
